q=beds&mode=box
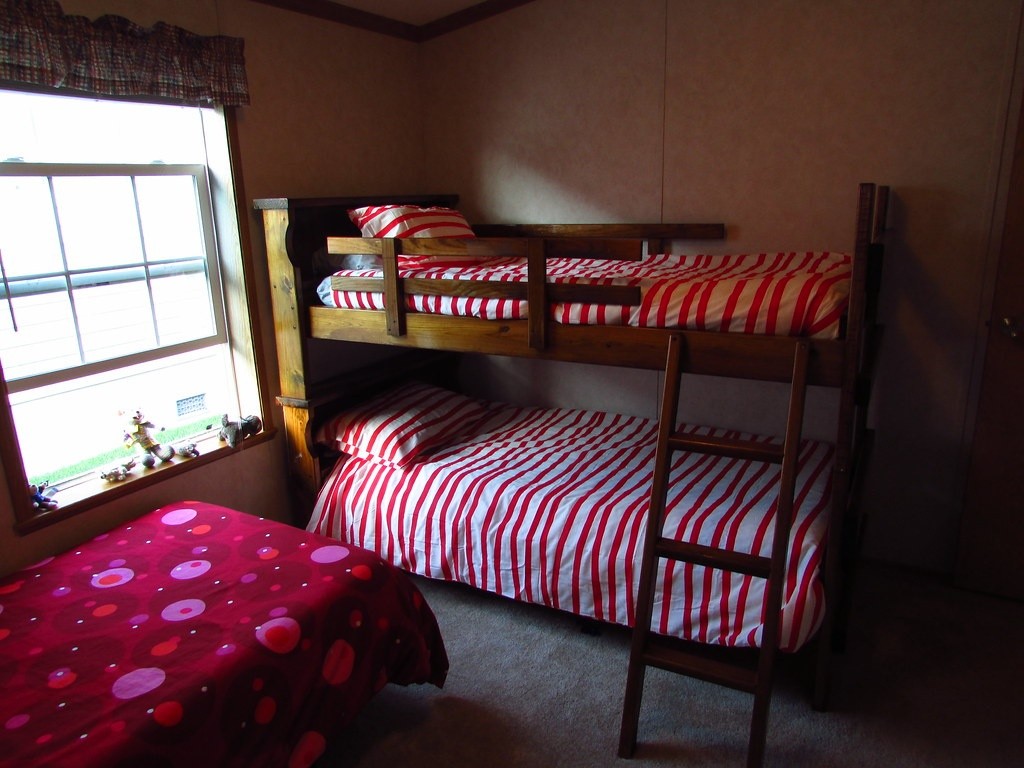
[254,191,892,374]
[273,390,836,652]
[0,500,451,768]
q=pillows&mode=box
[312,382,489,469]
[346,205,486,267]
